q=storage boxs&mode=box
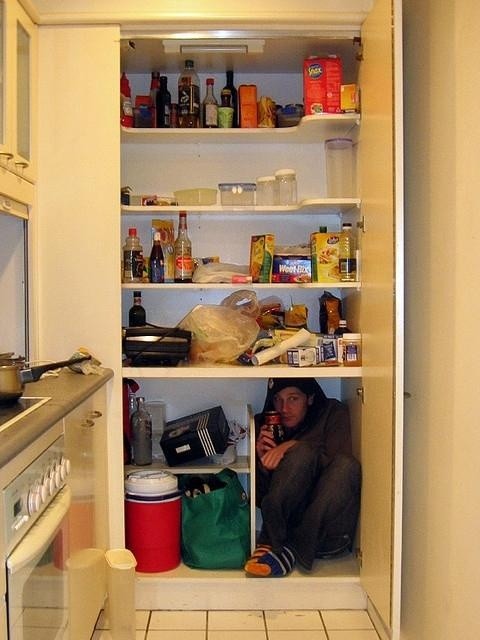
[160,405,233,468]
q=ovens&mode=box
[0,491,70,640]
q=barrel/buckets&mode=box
[124,470,182,574]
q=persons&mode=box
[243,378,362,579]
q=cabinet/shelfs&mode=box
[66,382,109,639]
[120,30,363,576]
[0,0,37,184]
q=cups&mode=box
[143,400,167,465]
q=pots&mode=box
[0,359,93,407]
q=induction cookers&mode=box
[0,395,71,548]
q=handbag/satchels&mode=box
[177,467,250,570]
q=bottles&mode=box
[334,320,352,334]
[149,59,239,128]
[338,222,356,281]
[122,212,192,283]
[129,392,153,467]
[275,169,298,205]
[323,138,354,198]
[257,176,279,205]
[120,71,135,128]
[129,290,146,327]
